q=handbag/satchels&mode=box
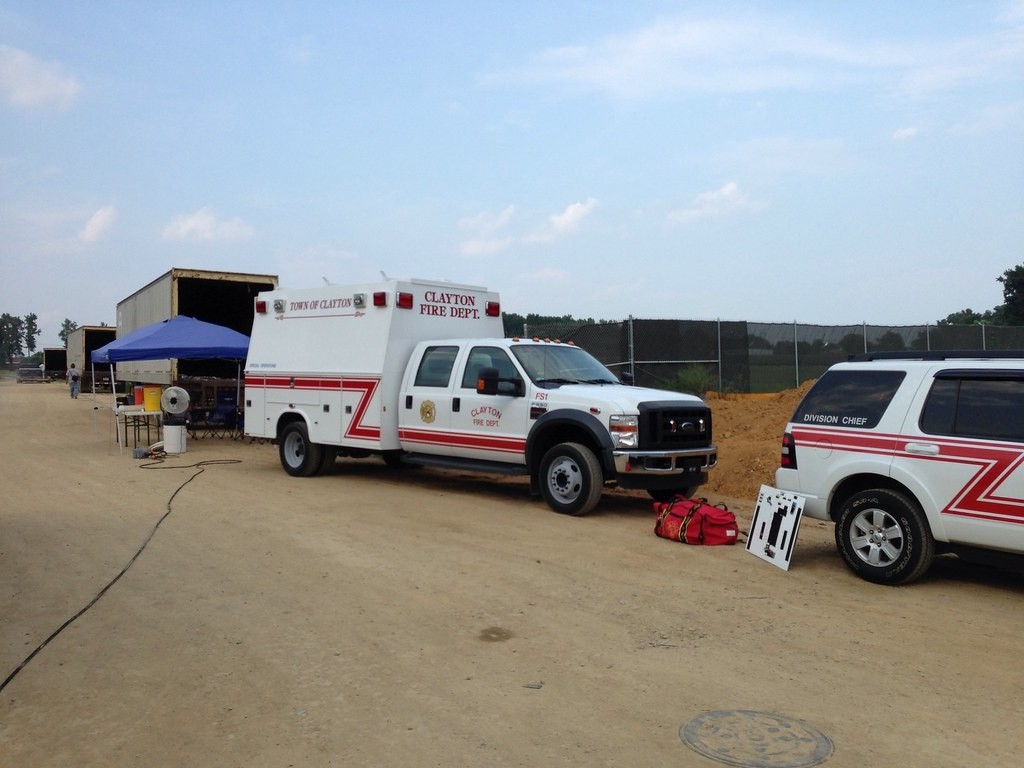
[72,375,78,382]
[653,493,738,545]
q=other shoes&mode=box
[74,395,77,399]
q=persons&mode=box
[38,362,45,378]
[65,363,80,399]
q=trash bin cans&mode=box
[163,418,186,454]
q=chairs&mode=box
[469,364,485,382]
[186,391,245,440]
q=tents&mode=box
[91,314,251,454]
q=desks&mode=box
[112,407,163,450]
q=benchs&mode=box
[427,359,511,389]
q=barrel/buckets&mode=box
[162,425,187,455]
[134,389,144,405]
[144,386,161,412]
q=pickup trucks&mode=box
[244,277,719,517]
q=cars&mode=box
[775,355,1024,587]
